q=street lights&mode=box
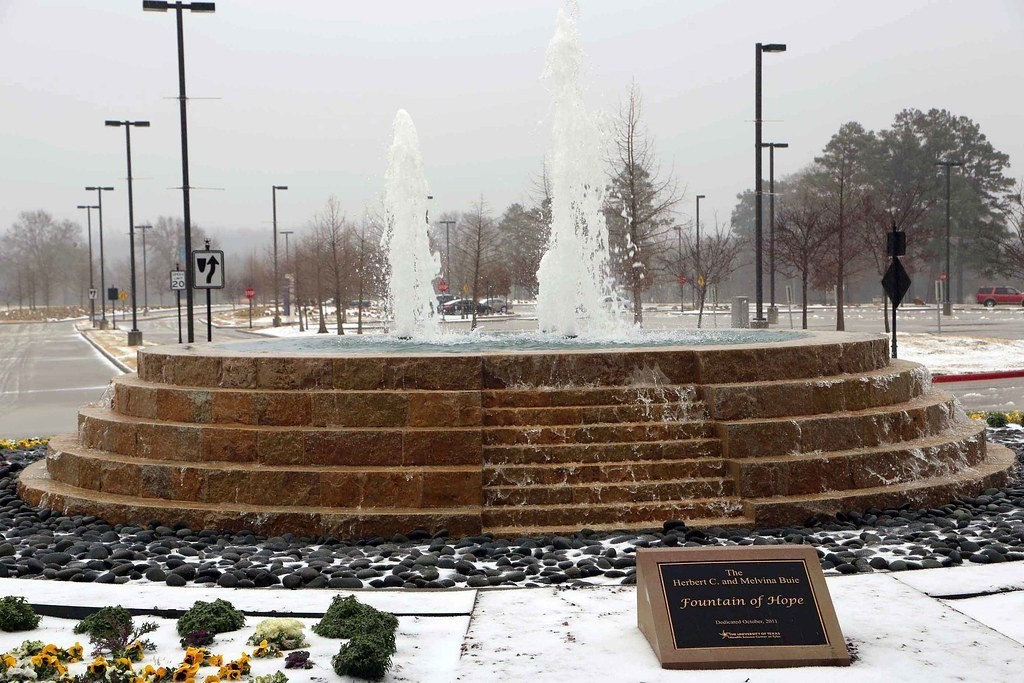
[85,187,115,328]
[104,120,150,347]
[279,231,294,266]
[695,194,706,276]
[76,204,99,326]
[134,226,155,315]
[760,141,790,322]
[935,161,962,316]
[439,219,457,280]
[272,185,288,326]
[754,43,787,320]
[674,226,684,312]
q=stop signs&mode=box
[245,288,256,299]
[438,280,450,292]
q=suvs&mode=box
[977,285,1024,307]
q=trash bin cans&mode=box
[731,295,749,329]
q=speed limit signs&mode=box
[170,269,186,290]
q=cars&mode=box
[434,294,510,315]
